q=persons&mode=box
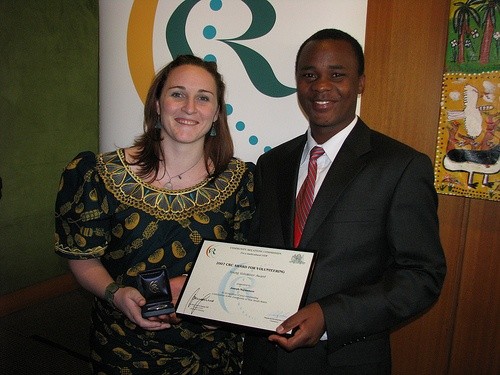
[54,54,263,375]
[242,29,447,375]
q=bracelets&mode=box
[103,281,126,312]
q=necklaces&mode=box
[160,151,203,189]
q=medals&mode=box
[147,304,168,311]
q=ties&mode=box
[293,147,326,248]
